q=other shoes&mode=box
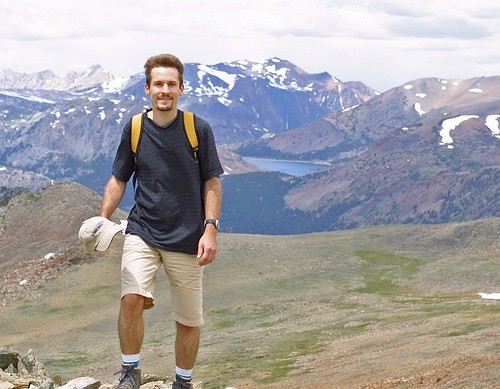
[114,368,141,389]
[172,379,193,389]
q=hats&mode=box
[78,216,124,253]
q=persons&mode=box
[79,54,225,389]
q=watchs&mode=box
[203,218,220,231]
